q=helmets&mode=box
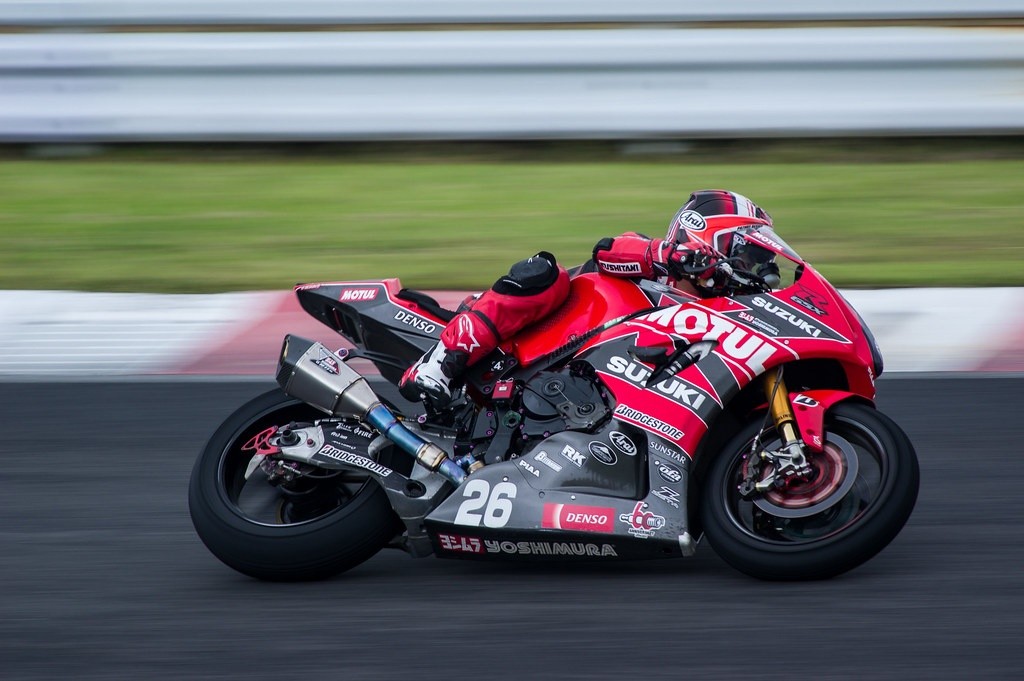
[666,189,804,297]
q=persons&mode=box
[399,189,775,403]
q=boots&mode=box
[398,309,504,408]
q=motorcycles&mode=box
[186,220,919,581]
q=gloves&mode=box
[651,239,718,279]
